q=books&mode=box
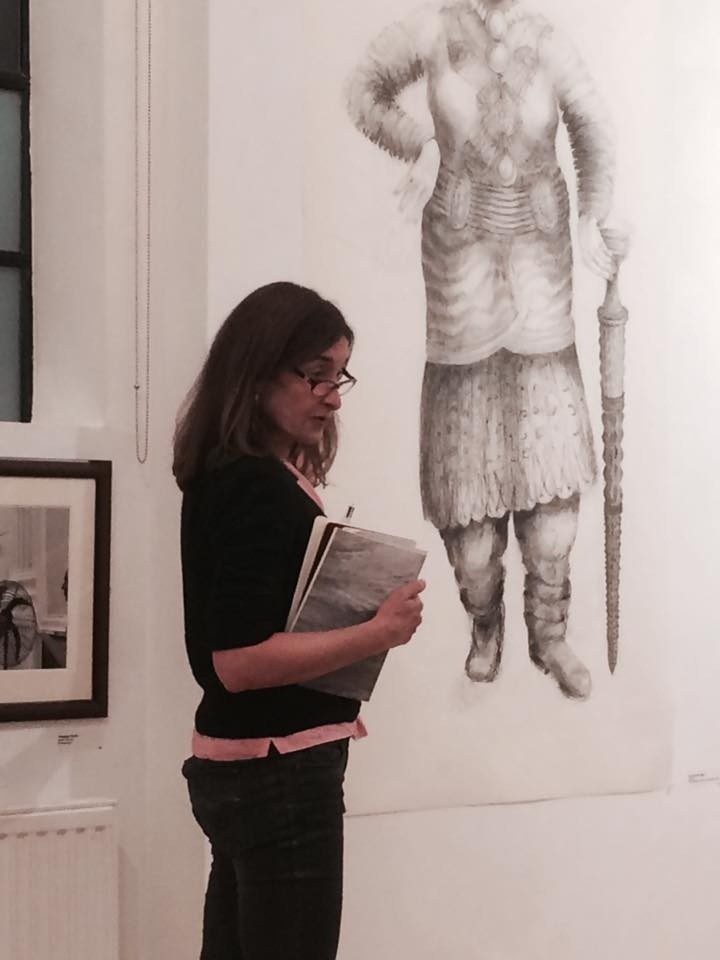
[285,515,428,702]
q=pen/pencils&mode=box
[344,505,355,523]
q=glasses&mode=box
[289,369,356,396]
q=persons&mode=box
[171,281,426,960]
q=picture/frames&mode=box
[0,460,113,722]
